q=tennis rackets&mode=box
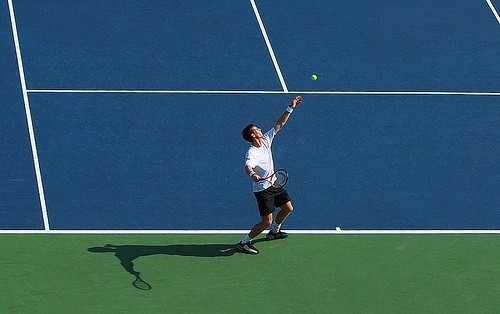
[259,169,288,189]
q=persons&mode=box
[235,95,302,254]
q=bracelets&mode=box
[249,171,256,177]
[286,105,294,113]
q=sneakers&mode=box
[235,240,259,254]
[266,230,288,240]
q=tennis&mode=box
[311,75,318,80]
